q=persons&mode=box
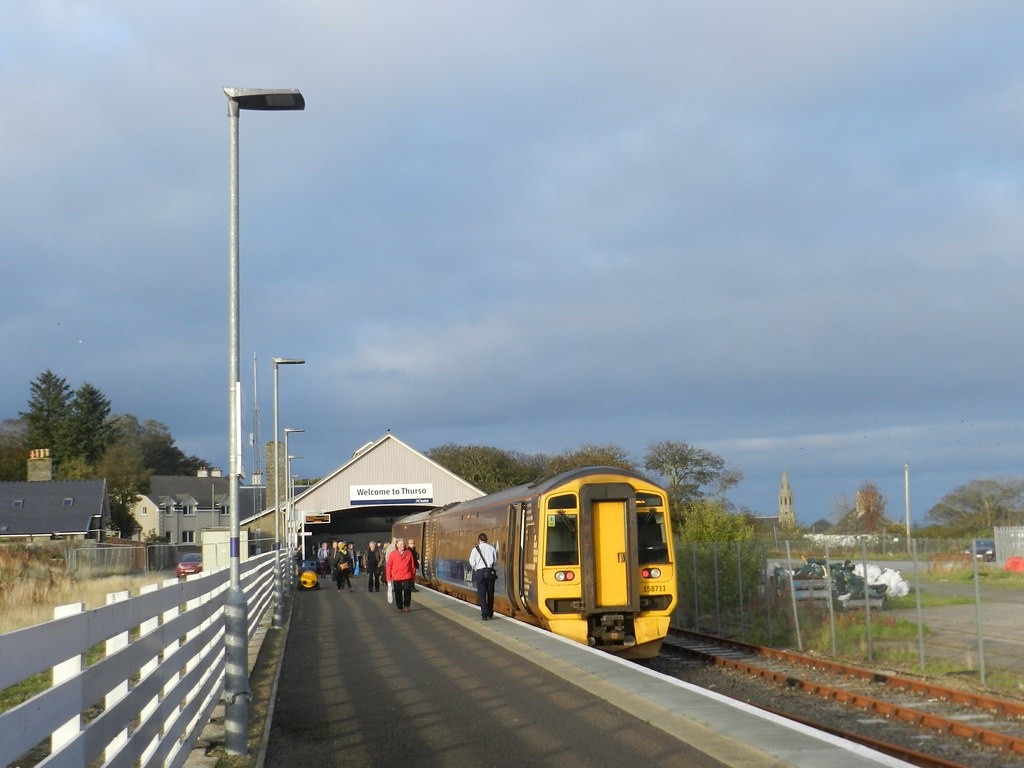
[317,538,420,612]
[469,533,497,620]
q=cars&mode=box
[175,553,203,578]
[962,538,996,562]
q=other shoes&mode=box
[481,613,490,620]
[350,588,356,592]
[400,607,403,612]
[338,589,346,592]
[376,587,379,592]
[411,589,420,592]
[404,606,409,612]
[369,587,372,592]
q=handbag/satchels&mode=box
[484,568,498,579]
[387,581,393,604]
[340,561,349,570]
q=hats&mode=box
[338,541,347,552]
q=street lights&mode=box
[221,86,309,758]
[287,455,304,589]
[271,355,306,625]
[282,428,306,598]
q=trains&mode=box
[391,463,680,660]
